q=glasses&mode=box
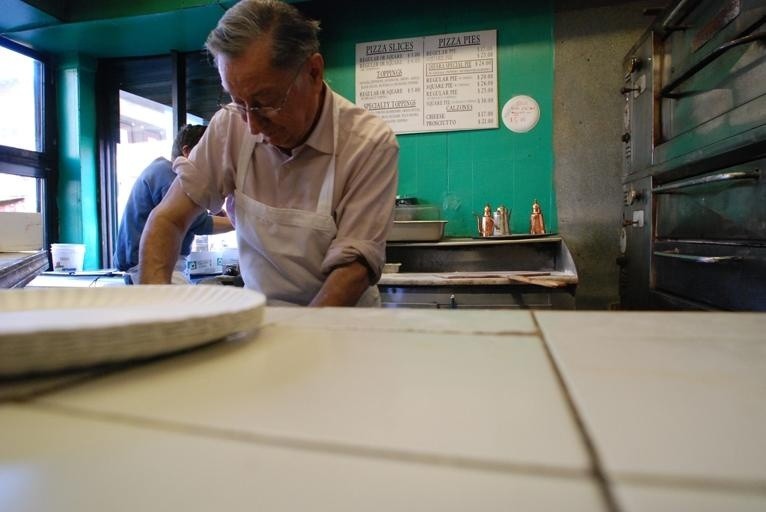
[217,60,307,118]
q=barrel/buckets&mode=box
[50,241,86,273]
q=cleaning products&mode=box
[531,197,545,233]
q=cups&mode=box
[50,243,86,272]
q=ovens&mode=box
[654,0,765,310]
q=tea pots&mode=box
[476,199,545,237]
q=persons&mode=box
[111,122,235,274]
[135,0,404,311]
